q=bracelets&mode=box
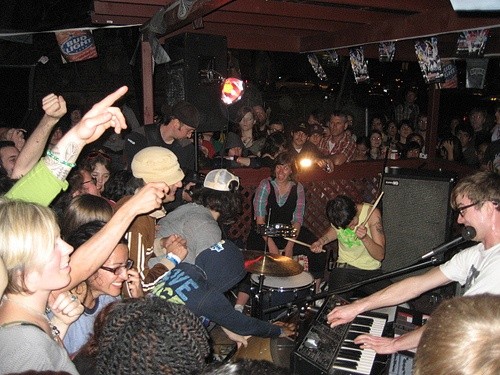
[168,251,182,263]
[165,254,178,266]
[234,155,239,162]
[47,149,75,167]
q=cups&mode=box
[390,166,401,175]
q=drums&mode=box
[250,274,316,320]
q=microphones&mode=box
[419,225,477,262]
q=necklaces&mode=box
[6,294,60,337]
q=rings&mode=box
[71,294,77,302]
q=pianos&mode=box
[292,294,391,375]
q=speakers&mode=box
[164,32,229,133]
[378,168,458,293]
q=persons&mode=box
[0,87,500,375]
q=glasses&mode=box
[82,178,97,184]
[420,119,429,124]
[457,198,484,216]
[371,136,380,141]
[84,151,112,165]
[101,258,134,275]
[347,119,355,123]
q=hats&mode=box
[131,146,185,187]
[293,120,310,135]
[162,100,202,128]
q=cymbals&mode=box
[244,252,304,277]
[261,225,297,239]
